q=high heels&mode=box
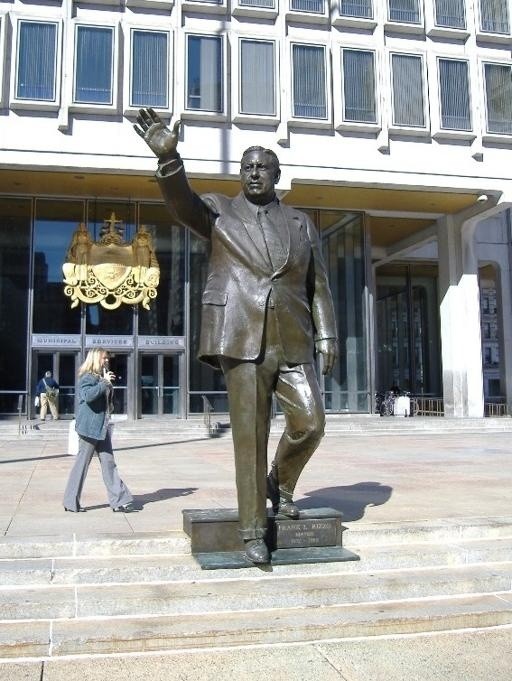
[113,506,131,513]
[64,506,85,512]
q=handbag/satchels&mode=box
[46,388,57,398]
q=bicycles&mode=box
[374,389,419,417]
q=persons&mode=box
[60,345,136,513]
[131,103,342,567]
[68,219,93,281]
[129,221,156,288]
[34,370,61,421]
